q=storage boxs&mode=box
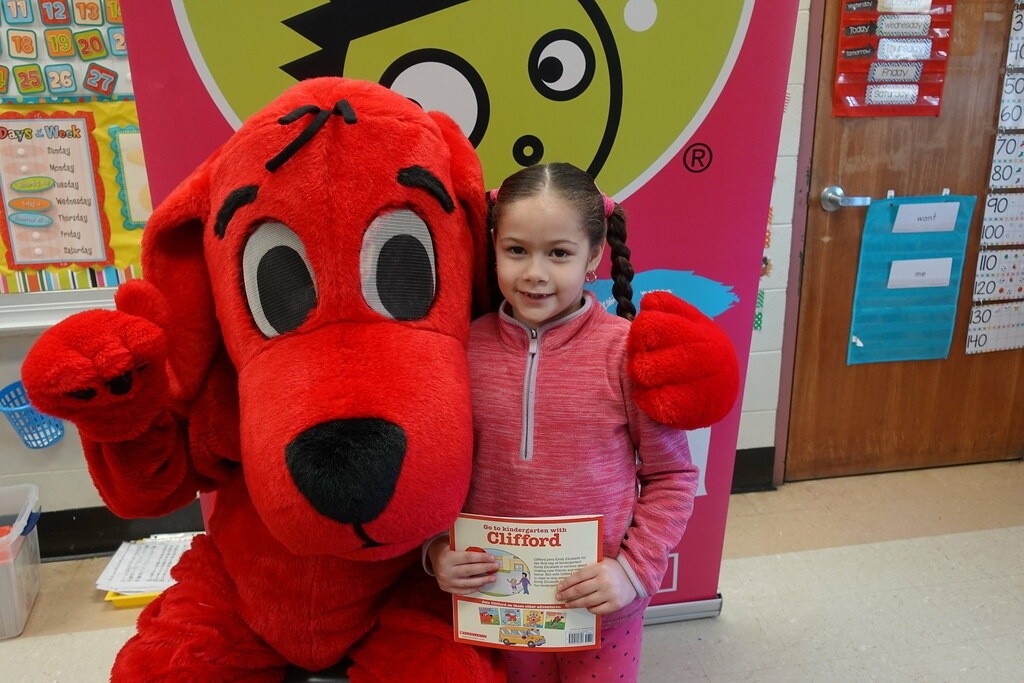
[0,483,40,641]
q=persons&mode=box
[420,163,699,683]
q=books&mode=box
[449,514,603,651]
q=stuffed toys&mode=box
[23,77,738,683]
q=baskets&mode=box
[0,379,65,450]
